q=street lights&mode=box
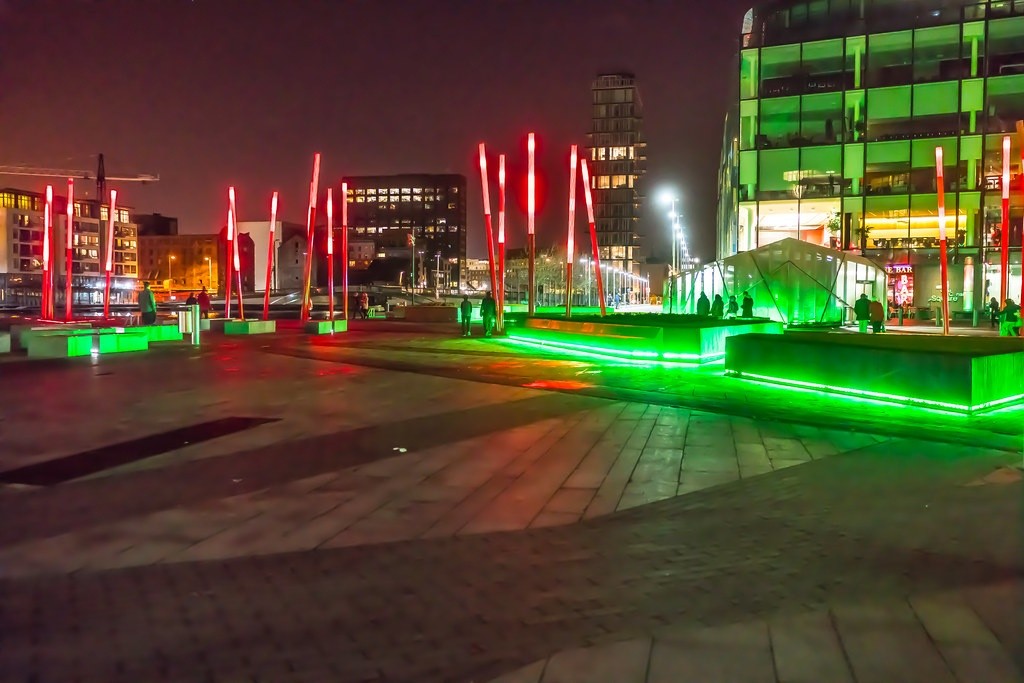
[168,255,176,301]
[204,256,211,289]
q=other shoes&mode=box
[485,330,489,336]
[489,333,492,336]
[468,332,471,335]
[461,333,466,336]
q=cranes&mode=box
[0,153,160,201]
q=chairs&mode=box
[873,233,967,247]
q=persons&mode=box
[710,294,724,320]
[989,297,999,328]
[724,296,738,318]
[697,292,710,317]
[461,295,472,337]
[352,291,369,319]
[740,291,753,317]
[855,293,870,333]
[868,295,884,334]
[608,291,620,310]
[197,287,214,320]
[997,299,1022,335]
[480,291,497,338]
[138,281,157,325]
[185,293,198,304]
